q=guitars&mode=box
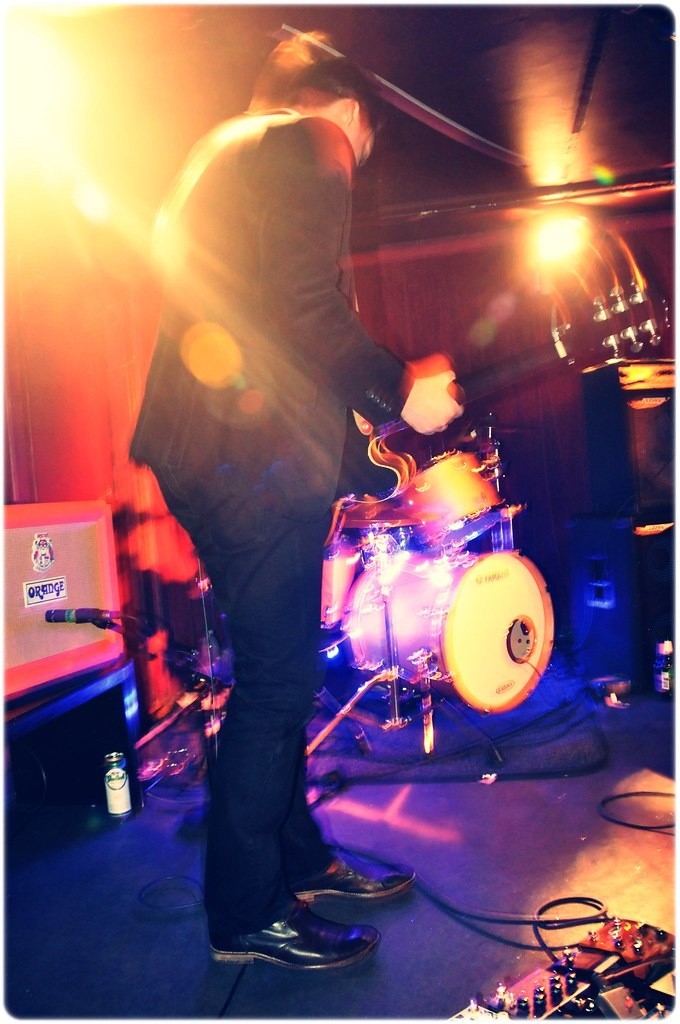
[330,276,664,504]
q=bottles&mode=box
[654,633,674,694]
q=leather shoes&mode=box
[209,899,381,971]
[293,848,416,903]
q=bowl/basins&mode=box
[588,675,632,698]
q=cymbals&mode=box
[334,493,425,528]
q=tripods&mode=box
[304,534,508,771]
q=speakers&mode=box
[566,510,674,694]
[584,390,673,516]
[4,500,126,703]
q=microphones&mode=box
[45,608,123,623]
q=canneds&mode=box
[102,751,132,817]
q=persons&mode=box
[128,30,464,971]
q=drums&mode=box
[320,533,362,630]
[338,545,555,717]
[389,449,508,555]
